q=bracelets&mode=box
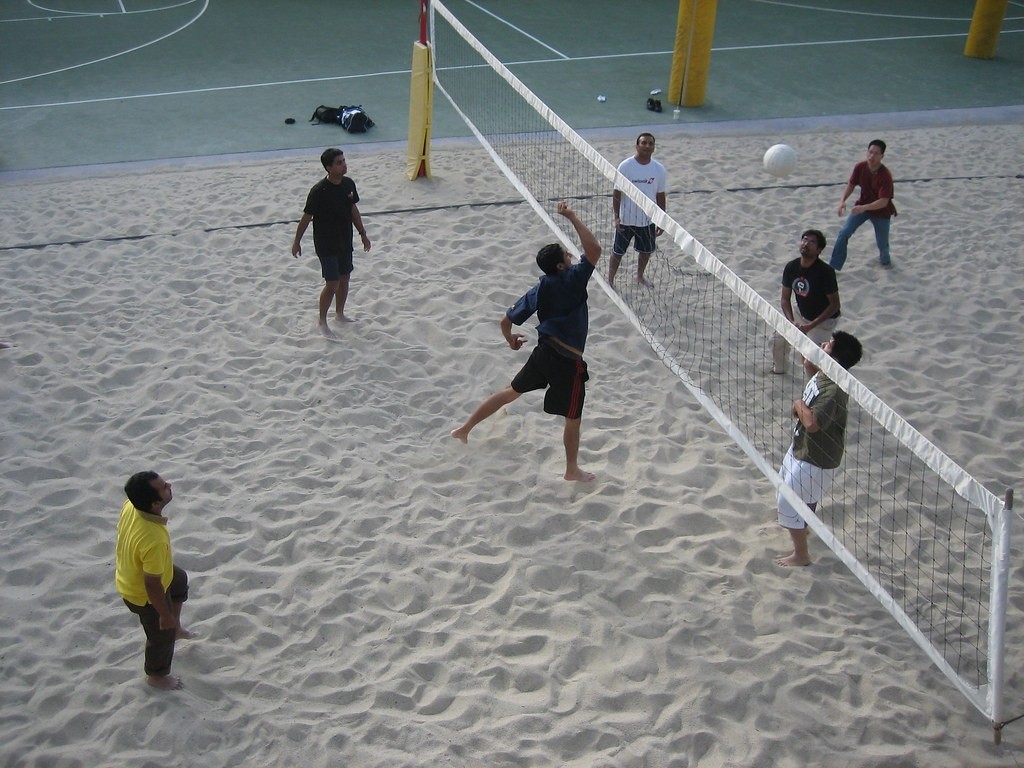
[359,229,366,236]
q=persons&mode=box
[776,331,863,567]
[292,148,371,340]
[768,230,841,375]
[452,202,602,482]
[115,471,200,692]
[830,139,897,271]
[608,133,666,290]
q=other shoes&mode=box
[655,100,662,112]
[647,98,655,110]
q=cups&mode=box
[673,106,681,120]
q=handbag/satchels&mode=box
[339,106,375,132]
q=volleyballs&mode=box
[763,143,797,178]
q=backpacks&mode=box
[308,105,337,126]
